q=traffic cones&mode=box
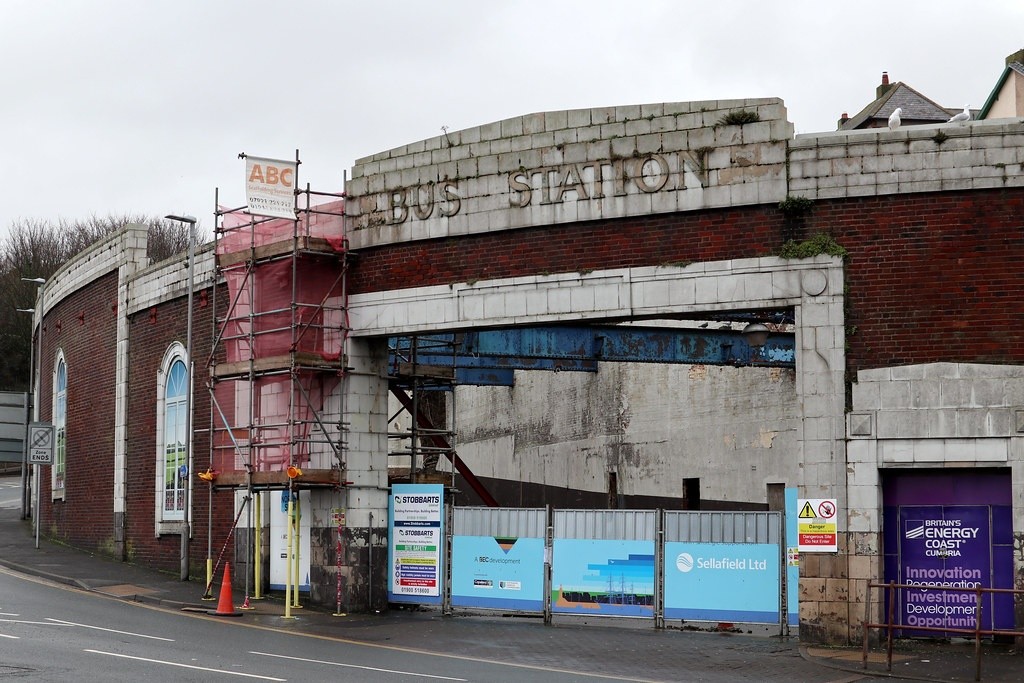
[207,561,243,617]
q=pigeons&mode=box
[888,107,903,130]
[948,104,972,126]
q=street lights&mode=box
[16,307,36,518]
[167,213,192,580]
[22,276,47,548]
